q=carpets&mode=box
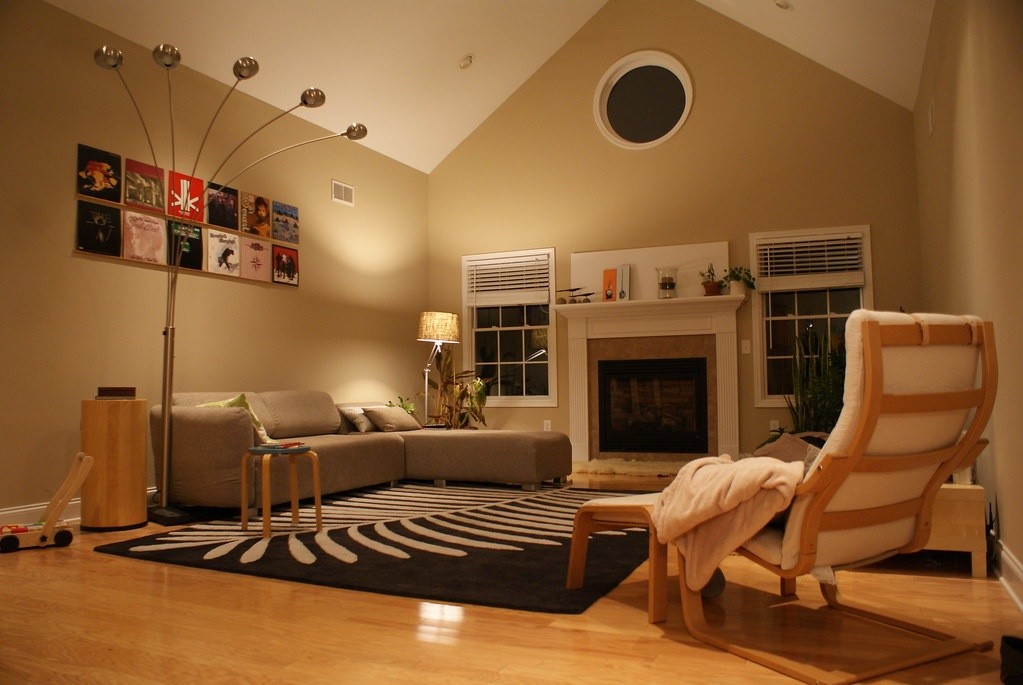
[93,478,650,616]
[573,457,688,476]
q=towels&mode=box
[651,452,804,592]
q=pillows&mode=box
[195,394,280,444]
[361,407,422,433]
[340,406,376,433]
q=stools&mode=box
[241,445,323,538]
[566,497,796,626]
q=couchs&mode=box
[149,390,573,519]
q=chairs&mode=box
[658,310,999,685]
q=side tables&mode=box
[78,400,148,530]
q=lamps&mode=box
[94,45,367,525]
[417,312,460,428]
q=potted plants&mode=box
[422,344,489,430]
[698,263,758,296]
